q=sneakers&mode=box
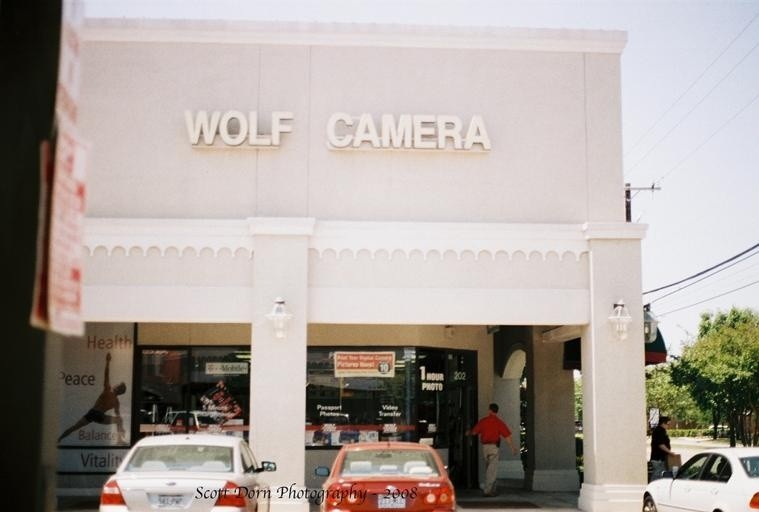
[484,492,495,496]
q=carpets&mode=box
[456,500,541,510]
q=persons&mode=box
[465,403,518,497]
[650,416,676,482]
[56,352,127,445]
[444,406,473,451]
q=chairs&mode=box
[410,467,433,474]
[200,462,226,471]
[379,463,397,472]
[140,459,164,470]
[713,458,729,479]
[403,459,424,467]
[348,459,372,473]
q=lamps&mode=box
[642,304,659,346]
[610,300,633,340]
[267,297,292,339]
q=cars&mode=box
[573,419,583,433]
[642,447,759,512]
[314,441,456,512]
[98,434,277,511]
[708,424,728,429]
[155,410,232,435]
